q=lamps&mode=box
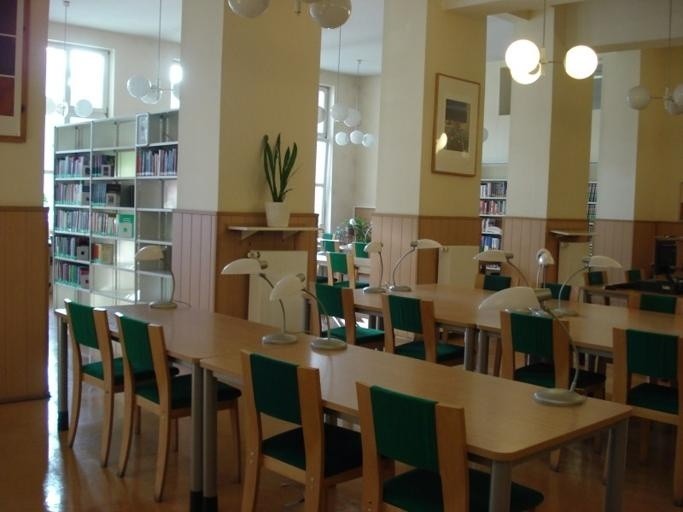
[334,58,376,148]
[46,2,93,117]
[626,0,683,117]
[534,248,554,288]
[473,250,530,286]
[363,241,386,294]
[134,248,179,310]
[268,273,348,351]
[316,28,362,129]
[219,258,297,344]
[477,286,587,406]
[388,238,442,293]
[550,255,622,317]
[126,0,181,104]
[503,0,599,86]
[226,0,353,30]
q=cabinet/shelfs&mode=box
[90,116,134,308]
[51,121,91,307]
[134,107,178,302]
[587,180,598,232]
[480,178,507,249]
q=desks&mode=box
[55,307,298,512]
[200,339,633,512]
[353,282,477,371]
[580,281,683,304]
[474,299,683,374]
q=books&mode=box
[56,155,115,178]
[138,147,177,176]
[56,181,121,205]
[481,182,506,197]
[481,218,501,234]
[481,236,499,252]
[480,200,506,214]
[54,262,89,286]
[57,210,116,236]
[54,237,82,259]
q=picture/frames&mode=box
[430,71,481,176]
[0,0,32,144]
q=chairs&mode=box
[540,283,581,303]
[353,379,467,512]
[582,270,607,285]
[63,297,180,468]
[113,311,243,504]
[627,291,683,315]
[474,274,521,292]
[624,268,644,282]
[496,309,603,473]
[602,327,683,503]
[239,348,362,511]
[381,293,465,367]
[307,281,385,351]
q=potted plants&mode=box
[260,132,303,226]
